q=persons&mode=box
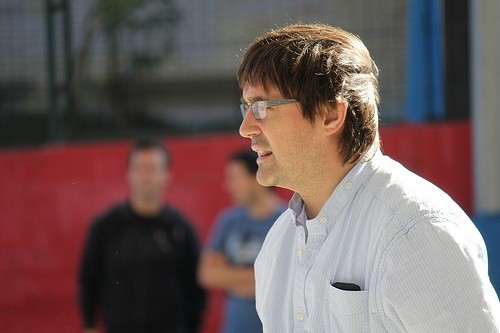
[237,22,500,333]
[195,148,289,333]
[77,138,210,333]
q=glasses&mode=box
[240,95,326,121]
